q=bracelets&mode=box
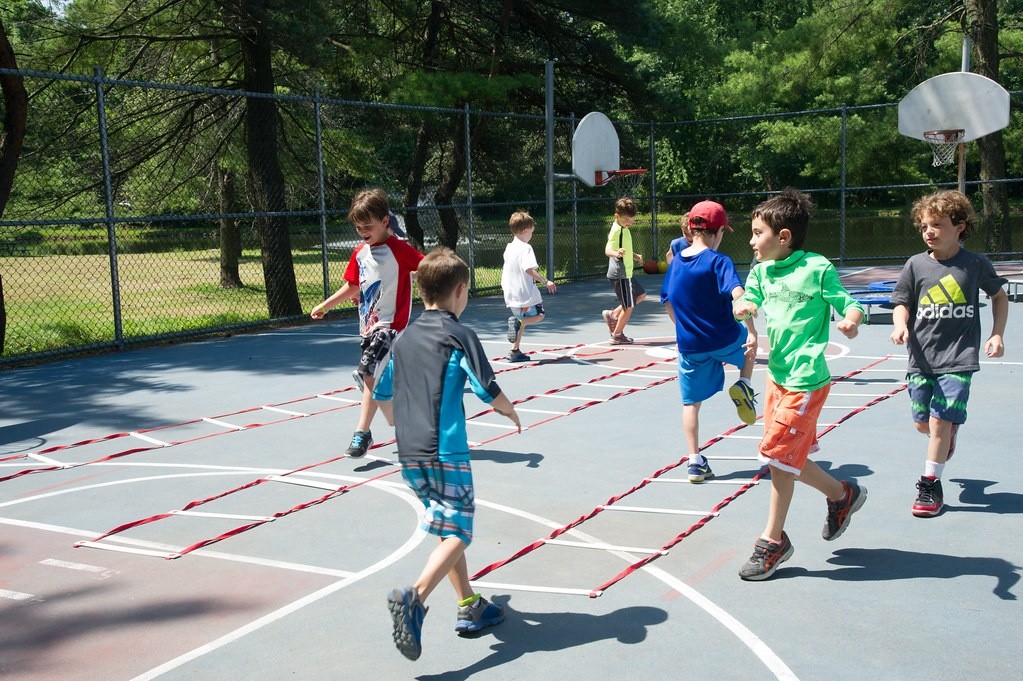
[318,305,328,314]
[543,279,548,285]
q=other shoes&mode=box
[352,369,364,391]
[506,316,520,344]
[507,349,531,363]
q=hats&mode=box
[687,200,735,233]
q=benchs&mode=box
[897,211,918,240]
[810,211,879,239]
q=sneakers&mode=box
[687,455,713,482]
[455,597,507,634]
[344,428,375,459]
[822,480,869,542]
[602,310,618,336]
[608,333,634,344]
[387,586,429,662]
[738,530,794,581]
[728,380,760,425]
[911,475,945,517]
[943,423,960,462]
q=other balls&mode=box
[656,259,668,273]
[644,259,659,274]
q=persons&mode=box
[889,190,1009,518]
[666,212,693,264]
[311,189,427,459]
[501,212,556,361]
[373,247,521,660]
[733,187,868,582]
[602,199,646,344]
[660,200,758,481]
